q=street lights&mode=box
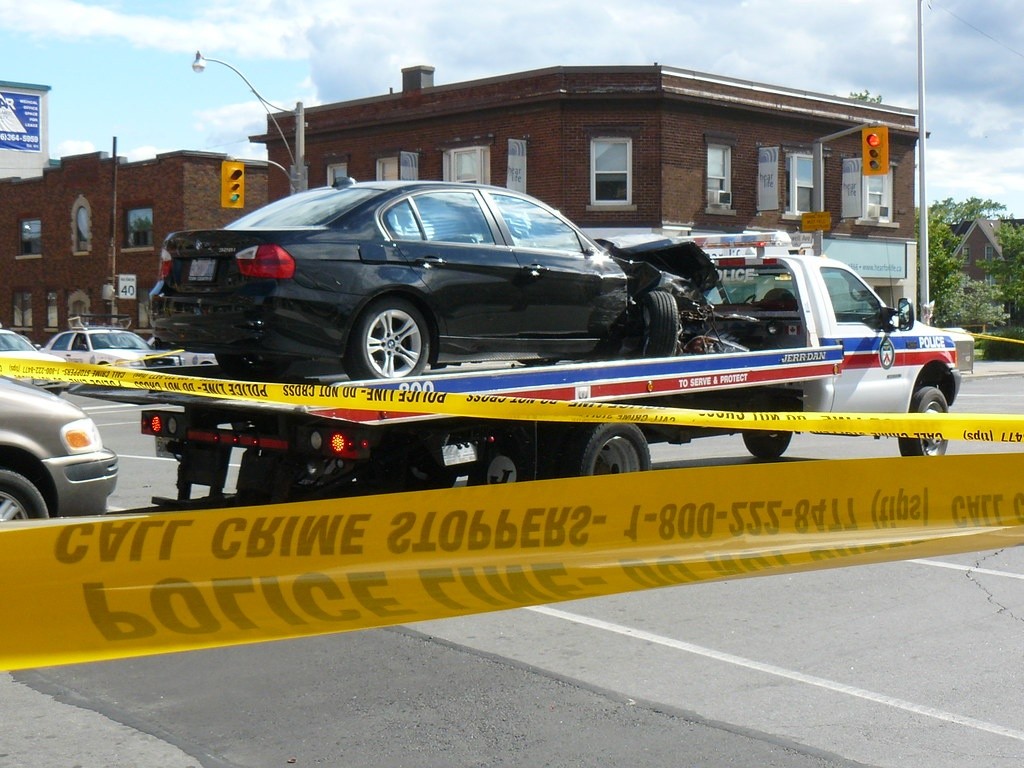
[192,53,308,195]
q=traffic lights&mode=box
[862,128,889,178]
[221,161,246,209]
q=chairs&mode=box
[52,339,121,350]
[762,287,799,312]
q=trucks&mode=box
[139,229,966,512]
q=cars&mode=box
[39,327,168,369]
[0,375,121,522]
[144,177,719,385]
[0,328,72,396]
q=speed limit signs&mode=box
[118,273,139,300]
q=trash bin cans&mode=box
[942,327,974,375]
